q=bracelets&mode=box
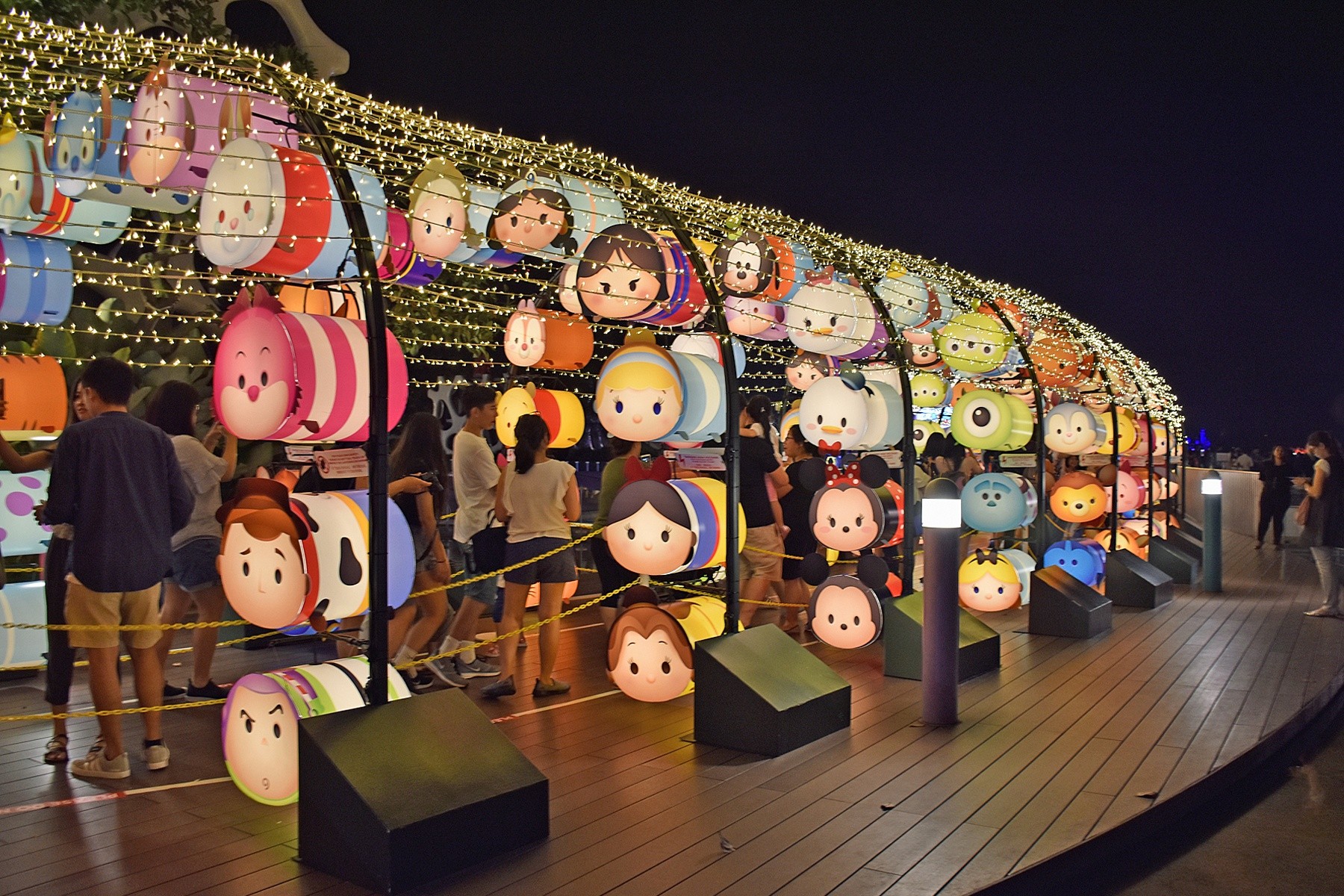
[1302,481,1308,489]
[437,560,447,563]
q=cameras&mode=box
[419,470,443,495]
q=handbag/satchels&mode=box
[1296,494,1311,525]
[472,508,509,573]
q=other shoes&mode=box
[519,632,528,647]
[1254,541,1265,550]
[805,623,813,633]
[780,622,801,634]
[1274,544,1282,552]
[533,676,571,695]
[485,643,501,657]
[480,673,516,697]
[779,524,791,540]
[1302,603,1344,616]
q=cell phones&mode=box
[0,378,5,415]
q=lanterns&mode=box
[214,276,416,806]
[709,228,1182,636]
[1,67,417,280]
[0,234,73,671]
[408,158,746,703]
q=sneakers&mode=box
[423,651,470,687]
[185,677,229,701]
[452,655,502,679]
[72,746,132,779]
[409,674,435,690]
[140,736,170,770]
[163,680,186,700]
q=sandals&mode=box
[88,733,107,753]
[45,734,70,764]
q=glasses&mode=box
[193,403,200,411]
[785,435,795,441]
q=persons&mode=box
[1189,447,1319,477]
[1290,431,1344,617]
[0,354,238,778]
[293,385,1111,700]
[1254,445,1293,549]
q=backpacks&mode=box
[941,455,966,495]
